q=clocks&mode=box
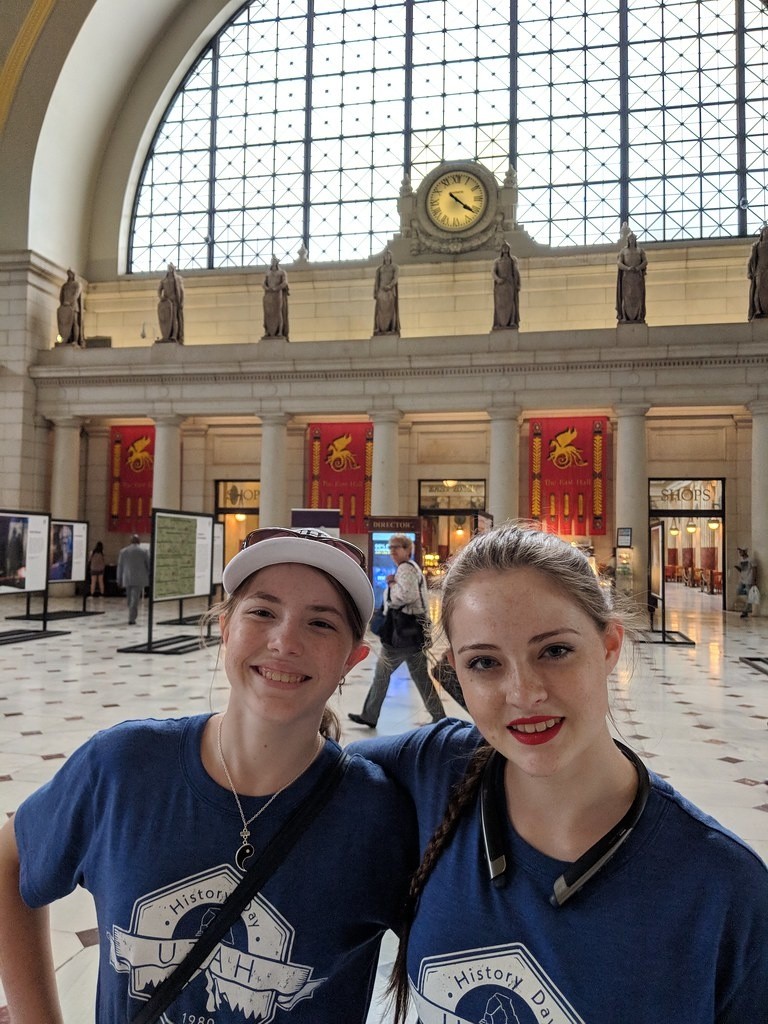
[415,162,499,241]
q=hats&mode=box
[223,528,375,633]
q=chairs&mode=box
[665,564,724,595]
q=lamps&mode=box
[670,496,721,535]
[230,484,248,522]
[455,515,466,537]
[444,480,459,488]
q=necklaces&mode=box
[217,716,324,872]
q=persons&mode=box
[699,568,707,592]
[347,535,445,726]
[734,545,760,618]
[0,527,418,1024]
[373,250,399,335]
[617,233,646,321]
[60,268,81,343]
[263,258,287,336]
[341,523,768,1024]
[88,541,106,596]
[492,243,520,327]
[116,534,150,625]
[746,226,768,316]
[158,262,185,342]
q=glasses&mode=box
[240,527,366,573]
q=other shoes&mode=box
[349,712,375,728]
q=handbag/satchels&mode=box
[371,606,432,649]
